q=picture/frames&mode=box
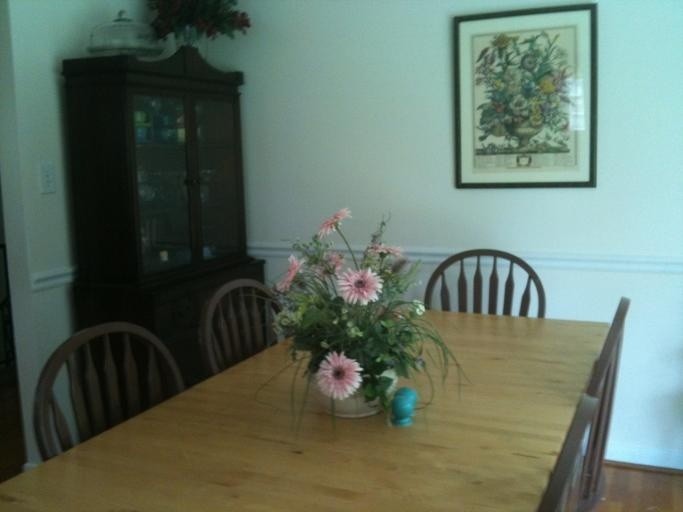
[451,4,598,188]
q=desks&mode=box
[0,307,608,511]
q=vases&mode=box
[317,369,398,420]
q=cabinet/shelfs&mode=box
[63,49,266,432]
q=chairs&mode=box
[541,396,601,511]
[423,250,546,318]
[589,297,630,508]
[199,283,289,378]
[34,324,183,461]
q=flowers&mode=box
[473,29,577,148]
[245,212,472,435]
